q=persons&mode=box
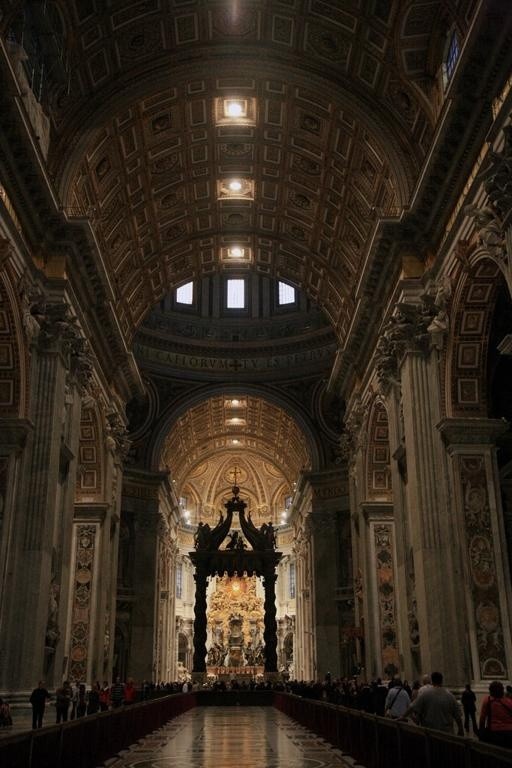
[29,680,52,729]
[213,671,464,737]
[505,685,512,699]
[461,684,478,734]
[55,679,200,725]
[478,681,512,751]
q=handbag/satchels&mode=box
[69,707,76,720]
[385,708,392,719]
